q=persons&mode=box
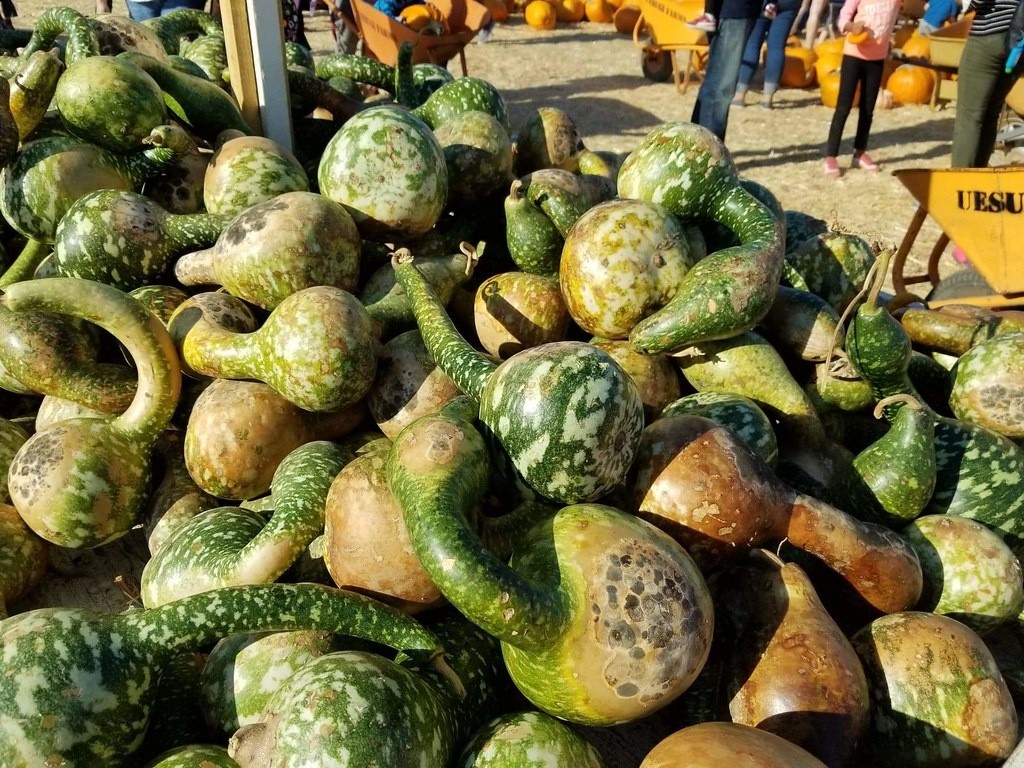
[823,1,902,176]
[126,0,363,56]
[952,0,1024,265]
[920,0,960,34]
[733,1,804,109]
[687,1,781,141]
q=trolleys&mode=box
[628,0,716,96]
[318,0,492,80]
[891,11,978,110]
[884,164,1024,331]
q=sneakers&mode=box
[826,156,842,176]
[852,153,878,171]
[687,16,716,32]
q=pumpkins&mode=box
[763,21,962,109]
[480,0,645,34]
[396,4,450,38]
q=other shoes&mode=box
[760,100,772,111]
[478,22,494,42]
[729,99,744,107]
